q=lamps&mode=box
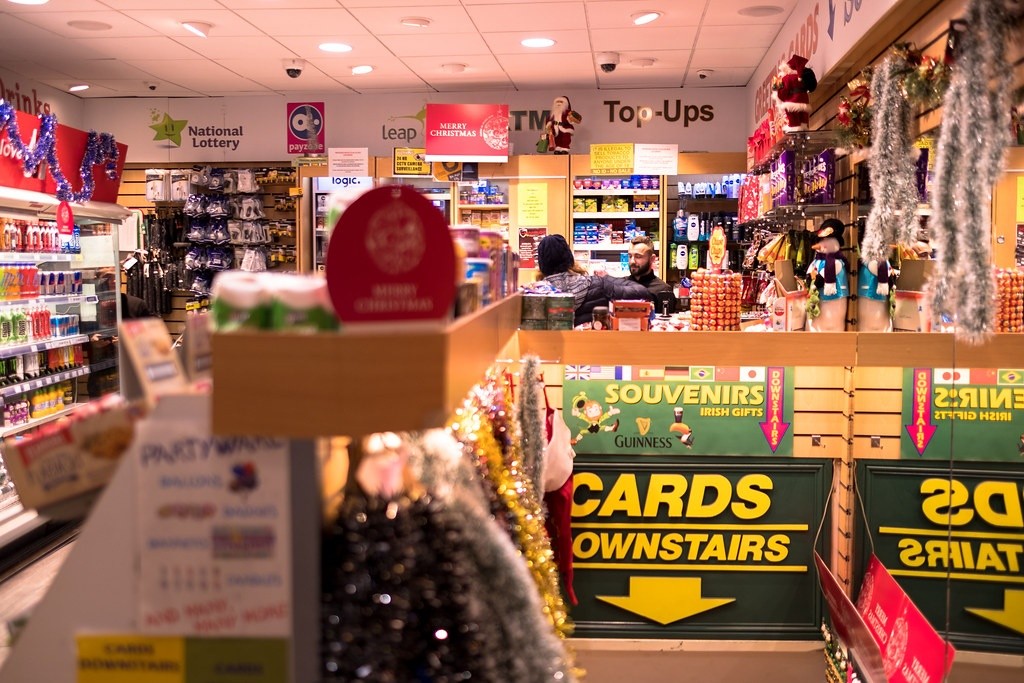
[68,85,89,93]
[442,64,466,72]
[632,11,659,26]
[631,59,653,67]
[181,22,215,37]
[401,18,432,27]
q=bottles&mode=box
[0,263,40,300]
[21,383,65,419]
[0,217,81,253]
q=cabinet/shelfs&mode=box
[0,185,132,585]
[297,152,746,285]
[748,131,849,332]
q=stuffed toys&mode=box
[772,54,817,132]
[858,219,901,332]
[536,96,582,155]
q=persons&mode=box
[87,267,150,405]
[594,236,677,314]
[805,218,851,332]
[536,233,650,327]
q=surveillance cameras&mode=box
[697,70,713,78]
[147,84,158,91]
[283,59,304,79]
[598,53,620,73]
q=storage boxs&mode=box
[608,298,652,331]
[892,258,936,332]
[522,292,575,332]
